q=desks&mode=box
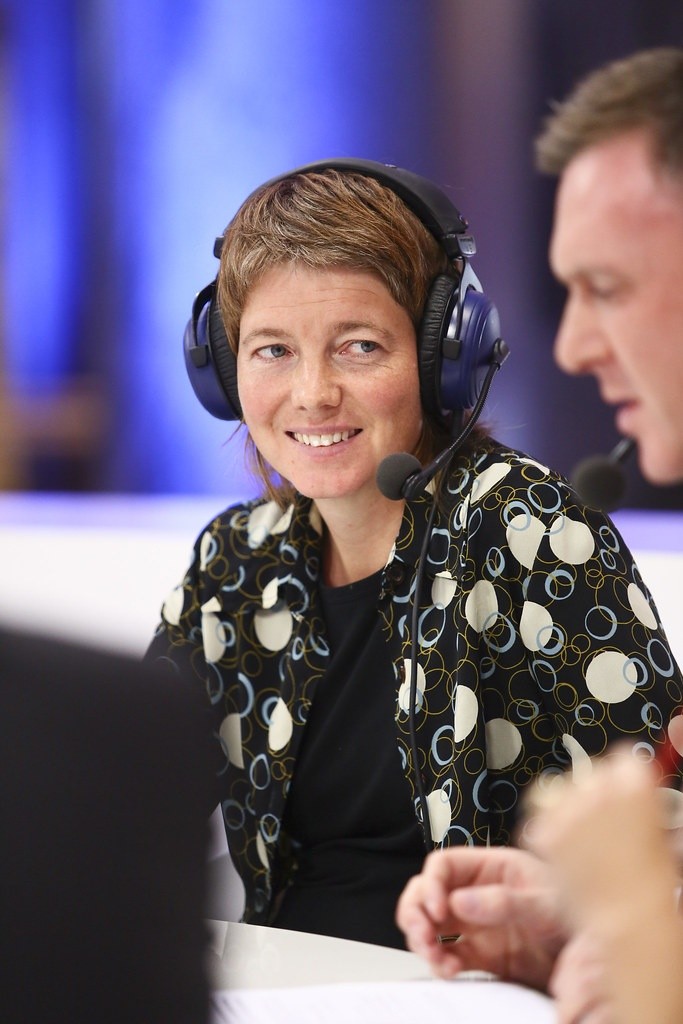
[201,915,559,1024]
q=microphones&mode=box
[376,338,511,502]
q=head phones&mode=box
[183,155,511,424]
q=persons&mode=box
[517,738,683,1024]
[392,47,683,1024]
[2,626,219,1024]
[145,153,682,959]
